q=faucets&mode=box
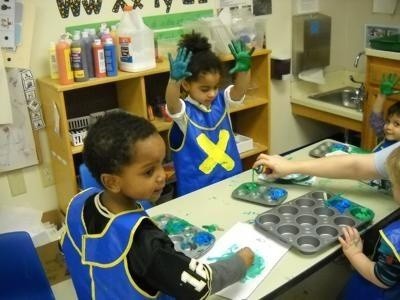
[353,51,365,68]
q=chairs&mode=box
[0,230,55,299]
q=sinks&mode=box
[307,86,366,109]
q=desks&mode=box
[147,136,399,300]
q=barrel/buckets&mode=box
[115,5,157,72]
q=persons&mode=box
[161,29,255,197]
[60,109,254,300]
[251,137,400,181]
[370,100,399,153]
[335,147,400,300]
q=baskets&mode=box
[67,116,89,146]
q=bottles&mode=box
[54,21,118,85]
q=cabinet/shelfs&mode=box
[32,48,274,219]
[361,56,399,148]
